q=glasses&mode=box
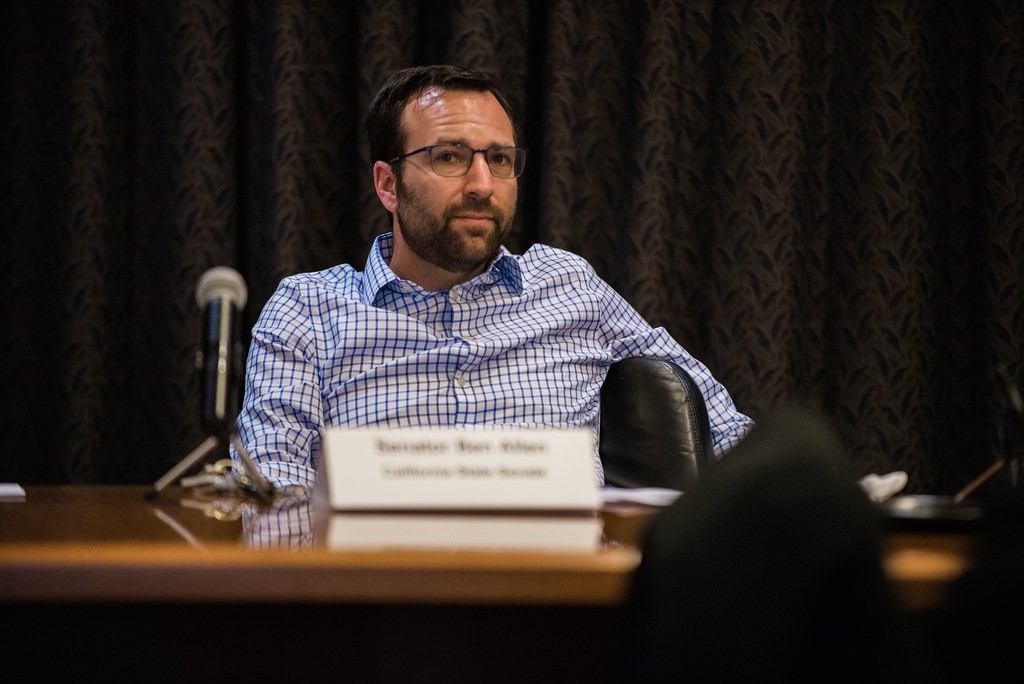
[385,142,527,180]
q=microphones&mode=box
[195,265,248,434]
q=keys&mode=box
[183,460,277,503]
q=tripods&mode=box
[144,430,275,501]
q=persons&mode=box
[229,64,756,490]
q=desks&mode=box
[0,482,963,607]
